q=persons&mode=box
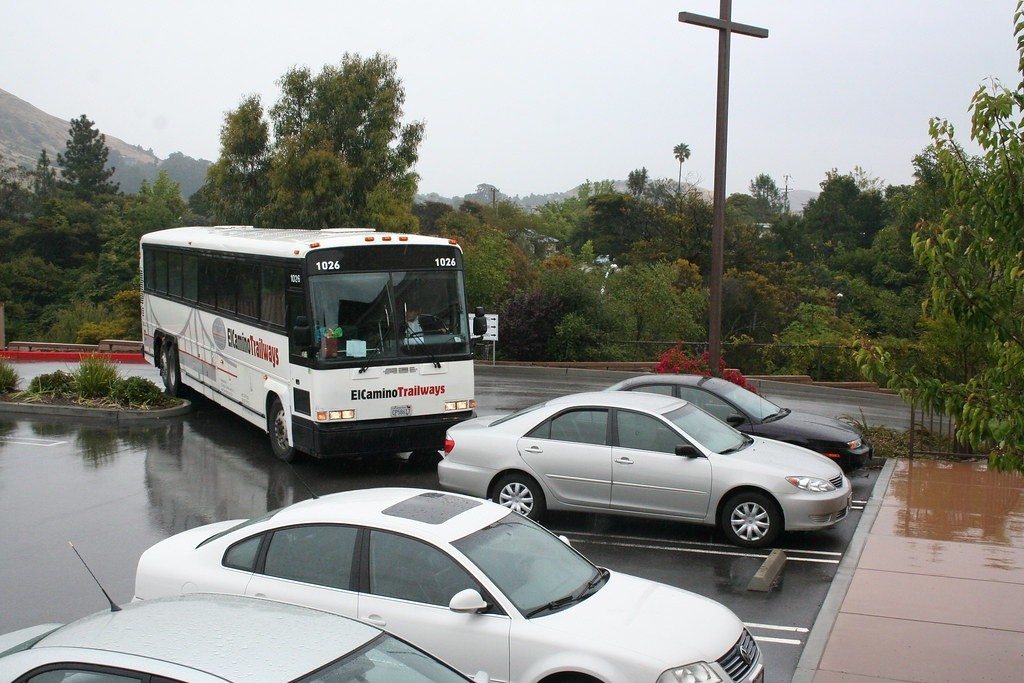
[398,303,446,340]
[339,317,358,338]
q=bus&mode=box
[137,224,487,464]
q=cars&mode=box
[126,487,765,683]
[0,593,480,683]
[436,390,852,528]
[577,375,874,472]
